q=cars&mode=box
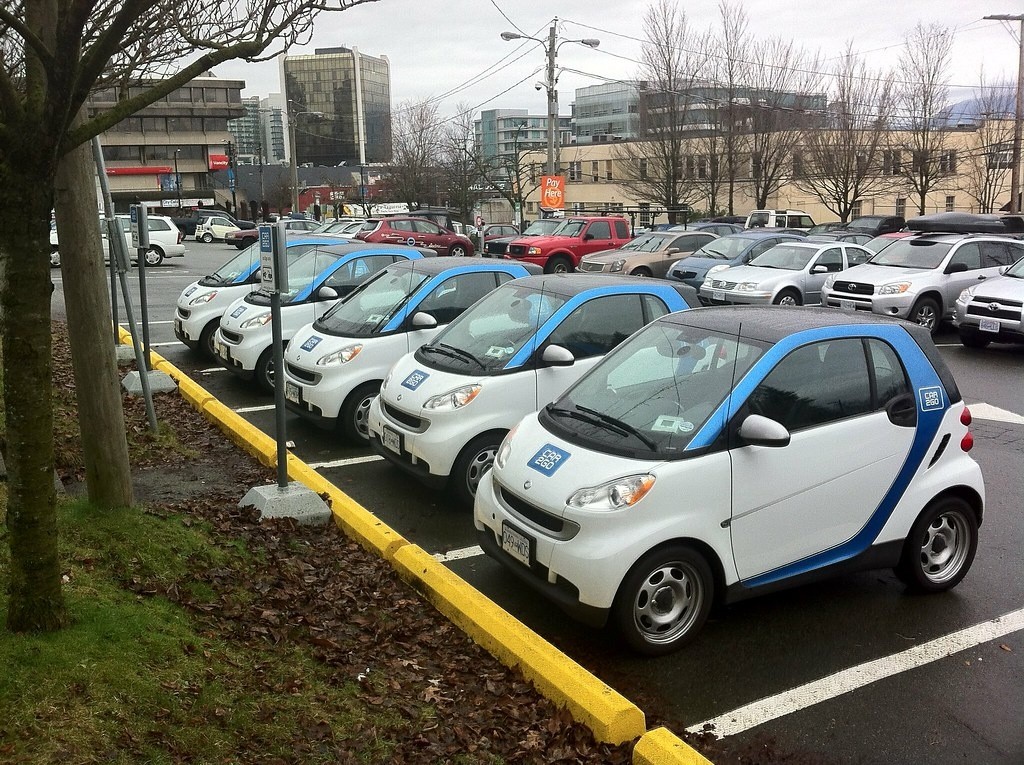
[366,272,726,514]
[453,208,910,285]
[225,208,439,250]
[353,216,475,258]
[213,243,457,397]
[952,255,1024,349]
[665,231,814,306]
[574,230,730,282]
[474,304,984,659]
[283,256,556,449]
[174,230,371,369]
[194,216,242,243]
[697,240,888,307]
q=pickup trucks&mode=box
[506,214,645,274]
[170,207,256,242]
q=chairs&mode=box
[325,261,350,286]
[565,309,620,359]
[759,334,877,394]
[794,252,816,268]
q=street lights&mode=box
[361,162,370,211]
[174,148,183,217]
[500,32,600,172]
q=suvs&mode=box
[820,212,1024,337]
[49,212,185,269]
